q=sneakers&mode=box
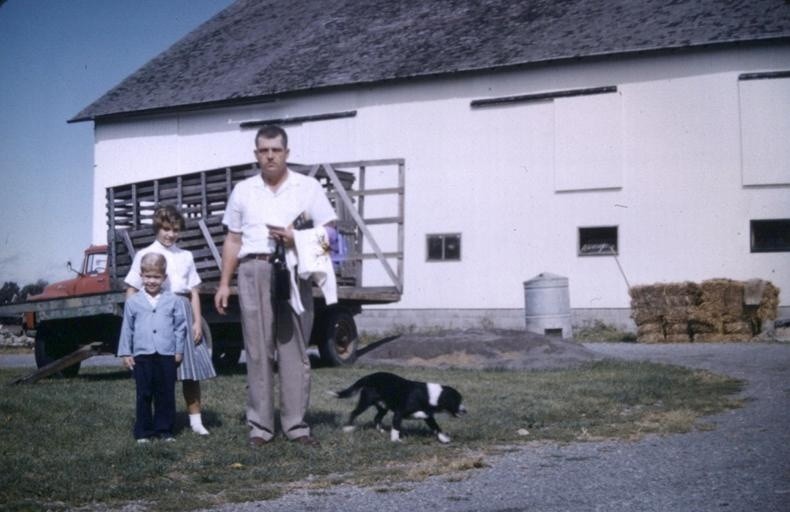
[159,430,177,441]
[137,438,151,443]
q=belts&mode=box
[245,254,273,261]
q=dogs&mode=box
[323,371,467,445]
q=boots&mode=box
[189,414,208,435]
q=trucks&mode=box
[23,157,408,379]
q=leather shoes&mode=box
[248,437,266,448]
[298,436,321,445]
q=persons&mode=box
[212,123,344,448]
[114,201,218,440]
[116,250,189,445]
